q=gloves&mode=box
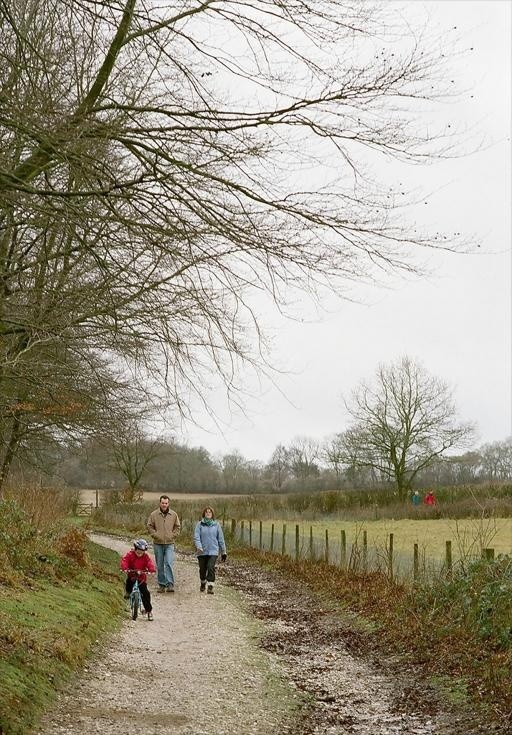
[221,553,227,562]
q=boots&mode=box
[200,581,214,594]
[156,583,175,593]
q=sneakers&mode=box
[148,611,153,621]
[124,592,130,601]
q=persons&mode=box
[423,488,436,505]
[412,490,420,505]
[120,539,154,621]
[193,506,227,594]
[147,495,181,593]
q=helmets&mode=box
[133,539,148,551]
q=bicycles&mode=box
[121,568,151,621]
